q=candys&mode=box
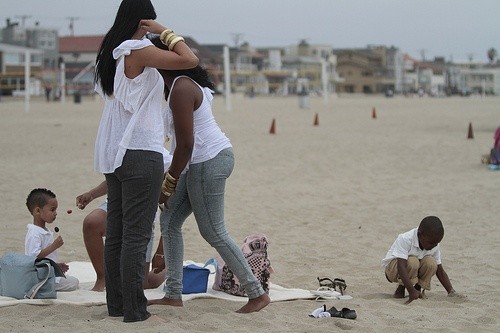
[55,227,59,236]
[66,207,78,214]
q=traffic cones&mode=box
[314,112,319,125]
[468,122,474,139]
[269,119,275,134]
[372,106,376,118]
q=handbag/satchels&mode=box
[0,252,66,299]
[217,231,273,296]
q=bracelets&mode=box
[160,29,184,51]
[161,170,179,196]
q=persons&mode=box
[94,0,200,322]
[146,35,270,313]
[25,188,78,291]
[383,216,467,304]
[76,147,174,291]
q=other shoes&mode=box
[333,278,347,292]
[335,307,356,318]
[317,276,334,289]
[327,307,339,317]
